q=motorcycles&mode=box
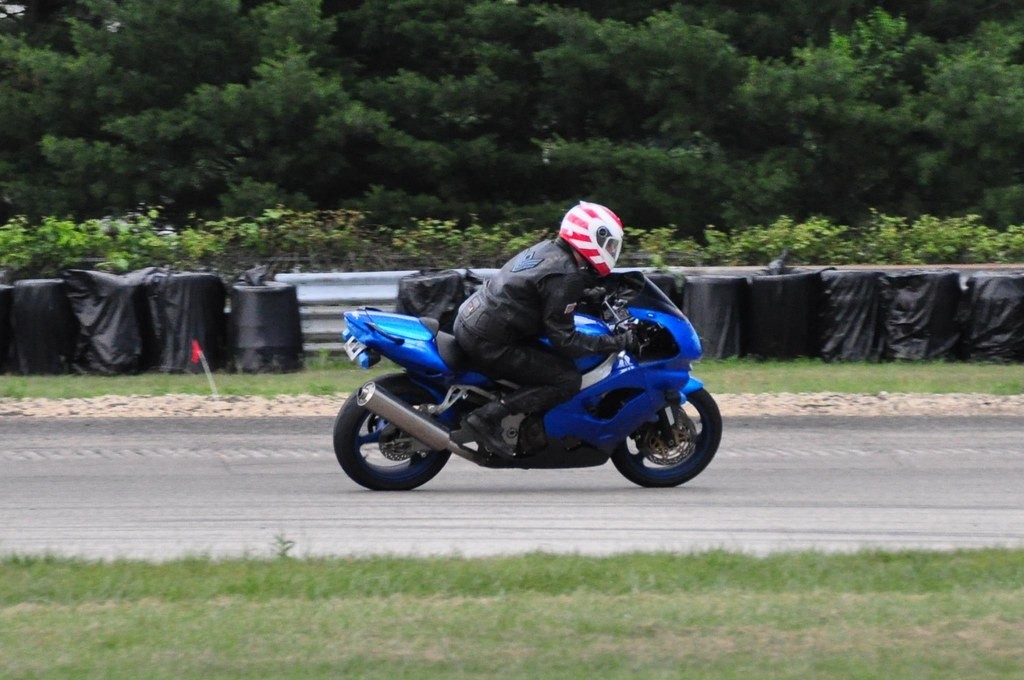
[334,270,723,492]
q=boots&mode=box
[460,398,531,460]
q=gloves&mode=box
[621,329,641,355]
[602,290,616,311]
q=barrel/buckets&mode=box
[231,281,304,374]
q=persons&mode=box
[453,202,643,459]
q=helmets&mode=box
[559,202,624,276]
